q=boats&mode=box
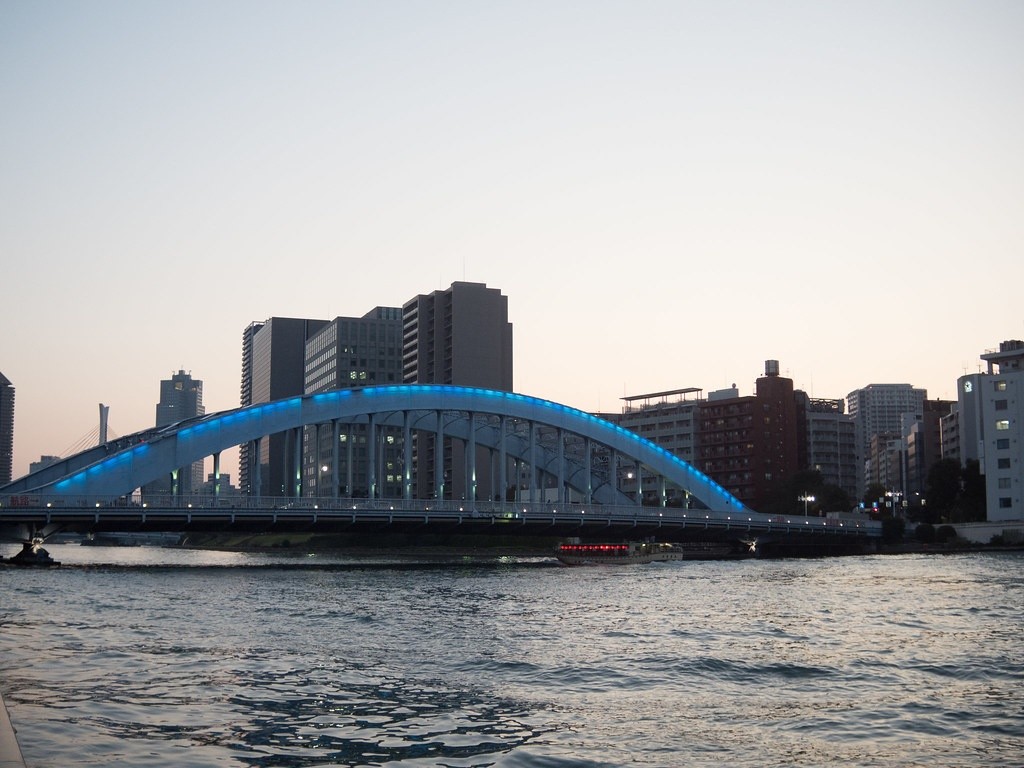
[552,538,686,566]
[91,532,181,547]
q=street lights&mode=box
[885,492,903,517]
[798,495,816,518]
